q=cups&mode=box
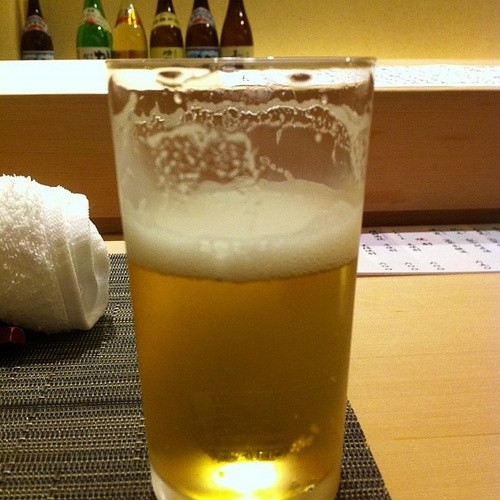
[104,57,377,500]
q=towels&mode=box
[0,173,111,334]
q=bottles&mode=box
[77,0,112,59]
[150,0,184,57]
[185,0,219,57]
[111,0,148,57]
[20,0,54,59]
[220,0,253,57]
